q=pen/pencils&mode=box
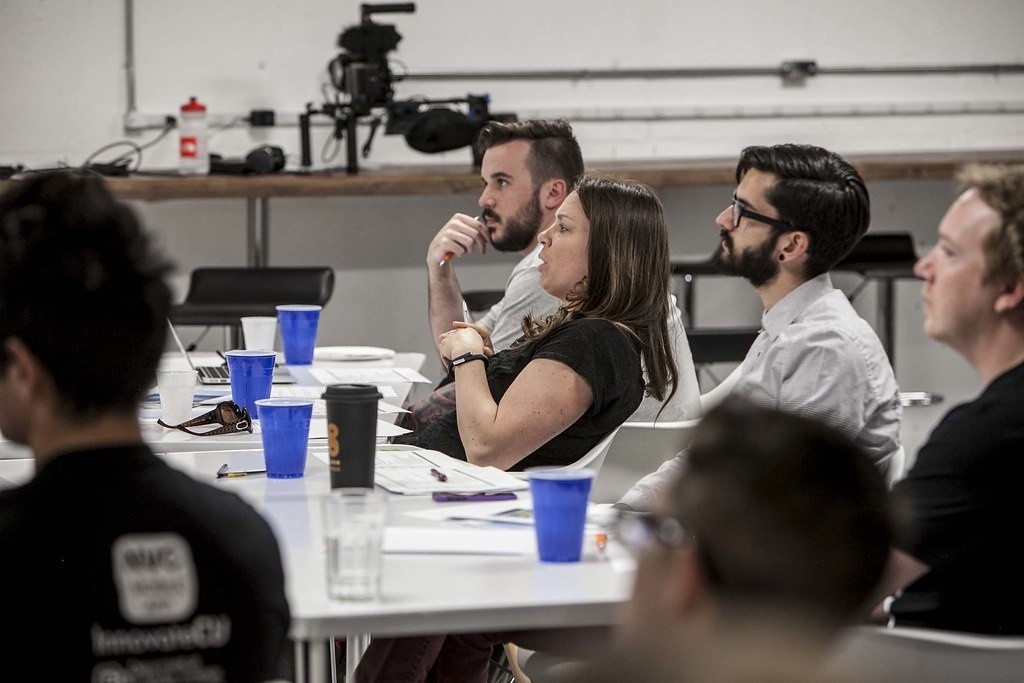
[218,464,228,475]
[439,216,480,267]
[218,470,267,478]
[431,468,447,482]
[462,300,471,325]
[598,533,608,554]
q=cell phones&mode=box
[433,493,516,502]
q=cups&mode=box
[156,370,198,424]
[527,466,595,563]
[321,486,385,600]
[321,383,383,488]
[255,398,316,479]
[276,304,322,365]
[225,350,276,419]
[240,316,278,350]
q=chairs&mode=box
[827,623,1023,683]
[591,419,705,499]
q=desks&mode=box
[0,351,651,682]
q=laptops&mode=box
[168,319,297,384]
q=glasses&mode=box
[731,190,795,230]
[612,506,722,596]
[157,400,253,437]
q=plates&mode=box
[313,346,396,362]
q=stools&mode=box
[658,258,768,393]
[851,234,926,368]
[156,266,334,349]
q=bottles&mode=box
[178,96,209,175]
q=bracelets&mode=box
[448,352,489,378]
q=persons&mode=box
[354,120,1024,683]
[0,164,292,683]
